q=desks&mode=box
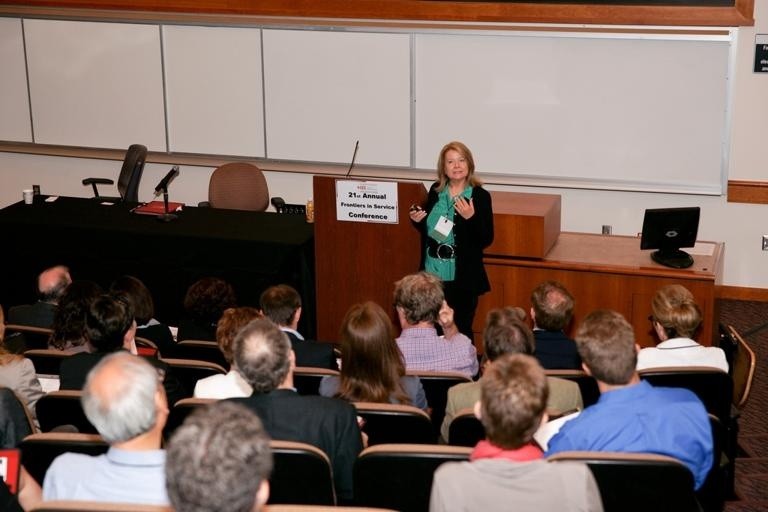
[472,232,724,360]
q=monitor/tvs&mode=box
[640,207,700,268]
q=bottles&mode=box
[304,197,314,223]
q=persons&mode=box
[194,308,264,400]
[428,352,604,512]
[0,387,34,512]
[633,284,729,373]
[40,350,170,505]
[530,280,583,370]
[2,266,237,432]
[392,271,479,378]
[226,318,369,506]
[542,310,713,491]
[165,401,272,512]
[2,321,78,433]
[260,285,339,371]
[317,302,431,414]
[406,141,495,345]
[441,305,583,445]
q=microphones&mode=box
[154,166,178,191]
[346,140,359,177]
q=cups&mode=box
[22,189,34,205]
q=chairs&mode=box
[0,325,732,511]
[91,144,147,209]
[197,161,285,216]
